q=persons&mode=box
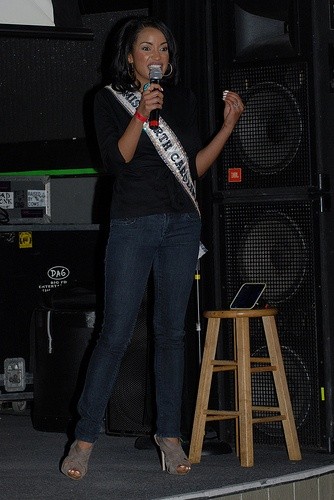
[62,16,243,478]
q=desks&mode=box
[0,222,232,456]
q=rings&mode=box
[233,103,239,107]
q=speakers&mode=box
[209,0,334,452]
[29,280,192,438]
[0,8,148,143]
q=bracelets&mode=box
[134,110,149,123]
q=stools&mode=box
[190,310,302,468]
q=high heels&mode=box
[61,440,94,480]
[154,434,192,474]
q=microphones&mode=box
[149,67,162,130]
